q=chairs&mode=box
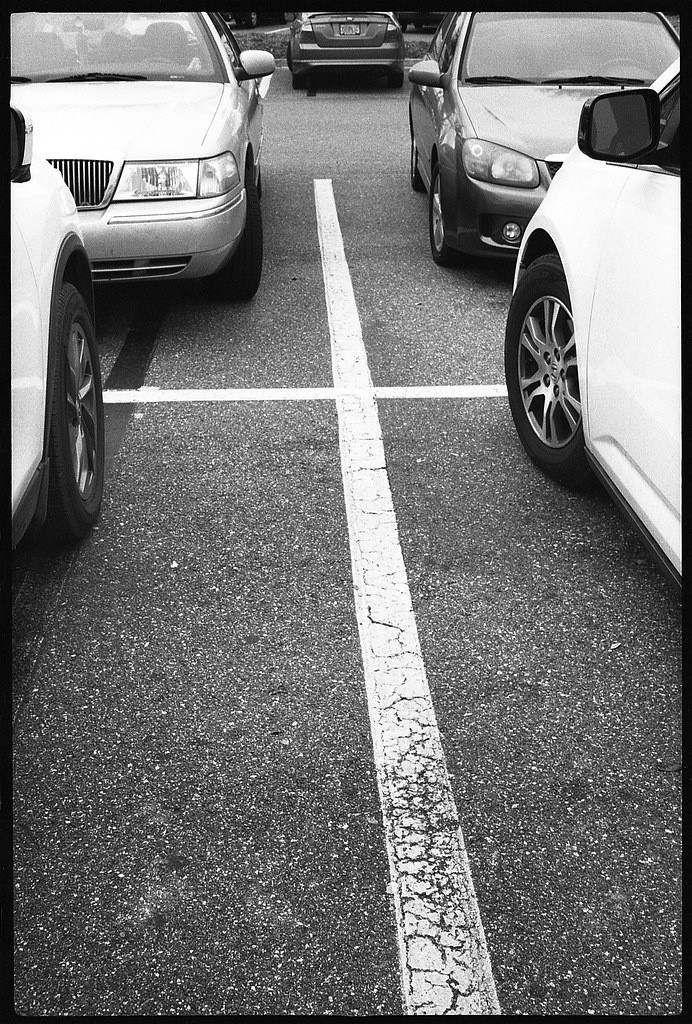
[129,22,205,72]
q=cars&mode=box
[8,108,110,556]
[405,10,682,263]
[284,12,407,89]
[503,56,684,584]
[10,11,278,306]
